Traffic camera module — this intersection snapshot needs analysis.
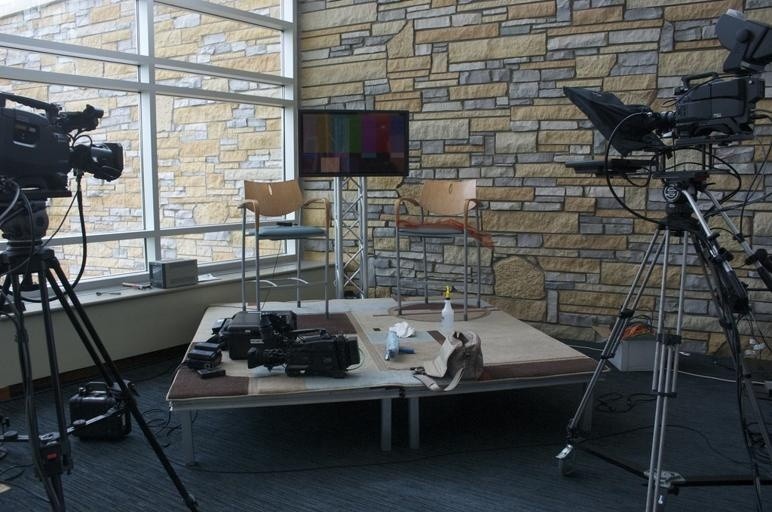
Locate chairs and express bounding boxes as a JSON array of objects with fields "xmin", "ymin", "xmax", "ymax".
[
  {"xmin": 238, "ymin": 179, "xmax": 331, "ymax": 319},
  {"xmin": 394, "ymin": 179, "xmax": 484, "ymax": 321}
]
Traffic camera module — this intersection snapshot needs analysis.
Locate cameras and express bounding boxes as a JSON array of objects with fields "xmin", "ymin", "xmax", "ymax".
[{"xmin": 188, "ymin": 342, "xmax": 223, "ymax": 370}]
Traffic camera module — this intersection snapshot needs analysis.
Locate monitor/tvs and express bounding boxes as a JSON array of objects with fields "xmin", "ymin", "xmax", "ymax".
[{"xmin": 298, "ymin": 109, "xmax": 410, "ymax": 178}]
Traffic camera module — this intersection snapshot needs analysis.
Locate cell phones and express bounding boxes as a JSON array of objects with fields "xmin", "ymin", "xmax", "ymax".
[{"xmin": 197, "ymin": 366, "xmax": 225, "ymax": 377}]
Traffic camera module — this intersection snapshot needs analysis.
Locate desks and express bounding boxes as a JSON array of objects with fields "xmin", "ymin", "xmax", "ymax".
[{"xmin": 165, "ymin": 293, "xmax": 610, "ymax": 451}]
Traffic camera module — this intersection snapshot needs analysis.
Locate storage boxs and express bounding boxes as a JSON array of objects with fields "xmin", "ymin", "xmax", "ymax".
[{"xmin": 591, "ymin": 324, "xmax": 665, "ymax": 372}]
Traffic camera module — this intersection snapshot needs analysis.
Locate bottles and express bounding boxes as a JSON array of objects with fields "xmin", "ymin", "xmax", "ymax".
[{"xmin": 442, "ymin": 298, "xmax": 455, "ymax": 334}]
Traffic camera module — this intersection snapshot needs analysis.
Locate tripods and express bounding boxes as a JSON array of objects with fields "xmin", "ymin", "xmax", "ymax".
[
  {"xmin": 0, "ymin": 246, "xmax": 199, "ymax": 512},
  {"xmin": 556, "ymin": 185, "xmax": 772, "ymax": 511}
]
